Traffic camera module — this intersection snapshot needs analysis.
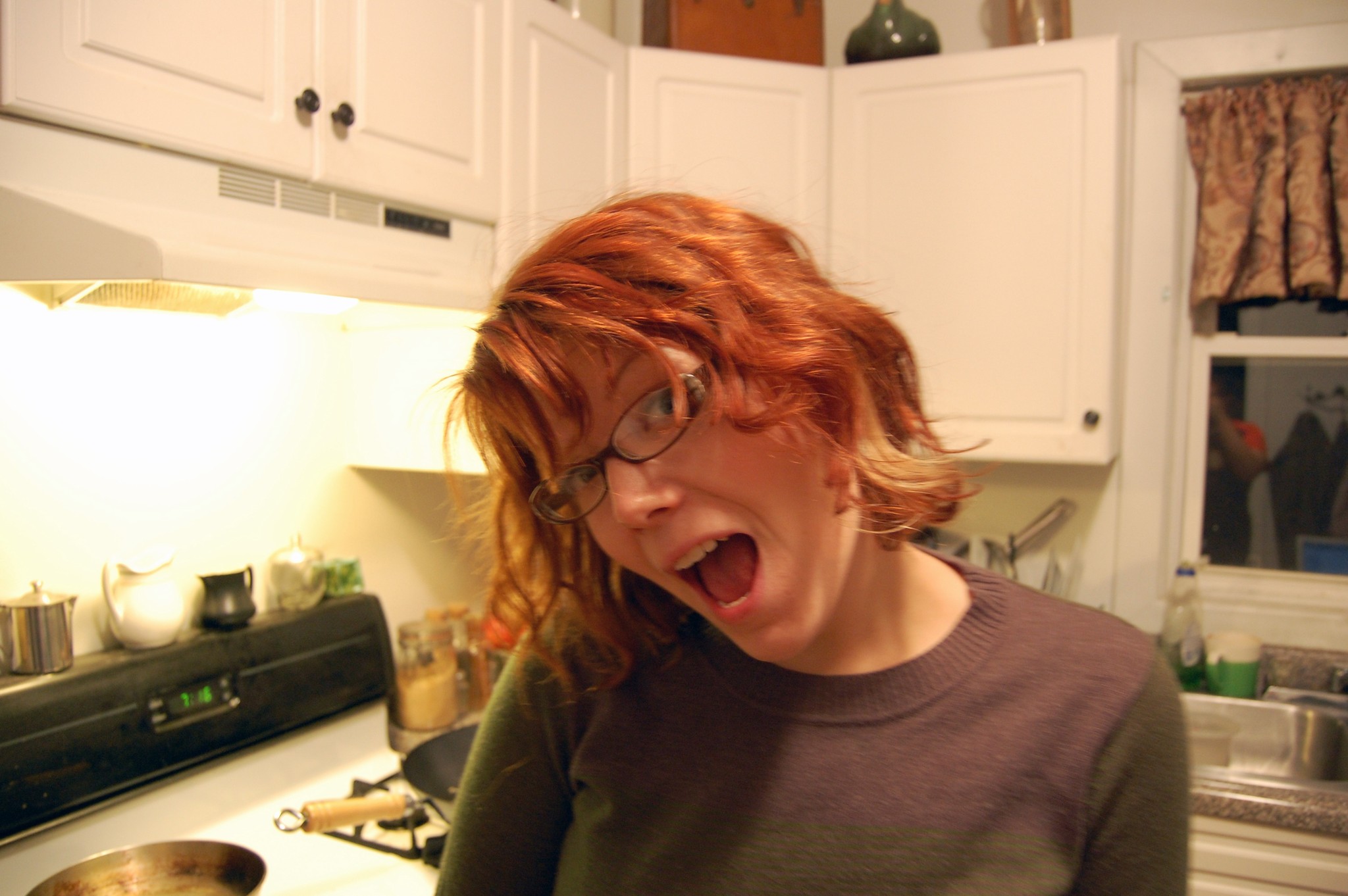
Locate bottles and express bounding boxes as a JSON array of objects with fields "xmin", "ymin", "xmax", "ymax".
[
  {"xmin": 846, "ymin": 0, "xmax": 941, "ymax": 65},
  {"xmin": 1159, "ymin": 564, "xmax": 1207, "ymax": 694}
]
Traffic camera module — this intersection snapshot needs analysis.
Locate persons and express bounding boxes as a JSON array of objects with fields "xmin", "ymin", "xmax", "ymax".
[{"xmin": 432, "ymin": 191, "xmax": 1193, "ymax": 896}]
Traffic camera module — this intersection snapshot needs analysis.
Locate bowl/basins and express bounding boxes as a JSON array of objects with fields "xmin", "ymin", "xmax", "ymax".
[{"xmin": 24, "ymin": 840, "xmax": 267, "ymax": 896}]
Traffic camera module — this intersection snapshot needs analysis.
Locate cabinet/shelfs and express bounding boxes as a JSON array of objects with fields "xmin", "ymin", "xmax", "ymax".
[
  {"xmin": 628, "ymin": 43, "xmax": 829, "ymax": 279},
  {"xmin": 1, "ymin": 1, "xmax": 500, "ymax": 225},
  {"xmin": 826, "ymin": 34, "xmax": 1109, "ymax": 469},
  {"xmin": 495, "ymin": 0, "xmax": 629, "ymax": 301}
]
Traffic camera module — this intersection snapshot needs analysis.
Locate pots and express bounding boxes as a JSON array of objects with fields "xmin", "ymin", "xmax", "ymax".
[{"xmin": 274, "ymin": 723, "xmax": 479, "ymax": 833}]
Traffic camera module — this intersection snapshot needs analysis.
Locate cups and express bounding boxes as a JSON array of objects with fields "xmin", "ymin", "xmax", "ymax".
[
  {"xmin": 1204, "ymin": 657, "xmax": 1263, "ymax": 701},
  {"xmin": 101, "ymin": 552, "xmax": 186, "ymax": 651},
  {"xmin": 1005, "ymin": 0, "xmax": 1072, "ymax": 45},
  {"xmin": 310, "ymin": 559, "xmax": 360, "ymax": 596},
  {"xmin": 199, "ymin": 564, "xmax": 257, "ymax": 629},
  {"xmin": 0, "ymin": 581, "xmax": 79, "ymax": 676}
]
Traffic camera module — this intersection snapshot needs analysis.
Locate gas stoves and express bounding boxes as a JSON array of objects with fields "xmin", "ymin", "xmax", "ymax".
[{"xmin": 0, "ymin": 591, "xmax": 451, "ymax": 896}]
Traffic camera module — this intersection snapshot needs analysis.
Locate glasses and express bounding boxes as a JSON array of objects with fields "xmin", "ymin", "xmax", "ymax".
[{"xmin": 529, "ymin": 356, "xmax": 725, "ymax": 527}]
